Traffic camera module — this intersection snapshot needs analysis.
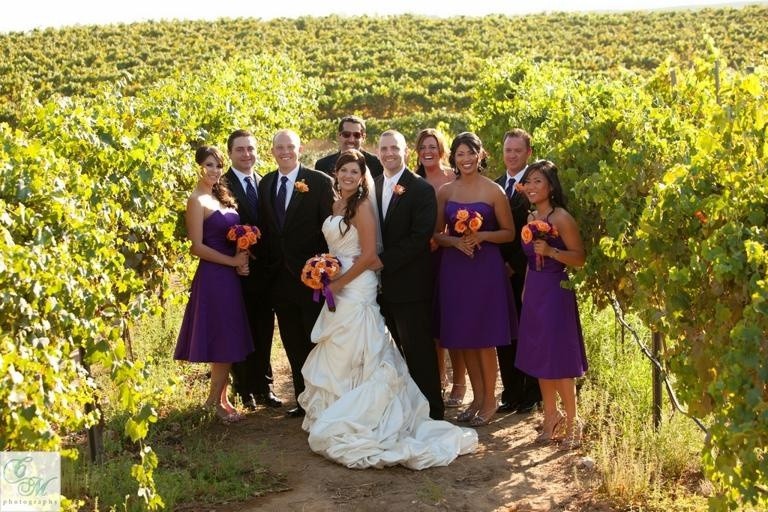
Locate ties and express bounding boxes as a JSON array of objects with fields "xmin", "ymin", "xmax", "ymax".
[
  {"xmin": 244, "ymin": 177, "xmax": 258, "ymax": 221},
  {"xmin": 276, "ymin": 176, "xmax": 288, "ymax": 230},
  {"xmin": 505, "ymin": 178, "xmax": 516, "ymax": 198}
]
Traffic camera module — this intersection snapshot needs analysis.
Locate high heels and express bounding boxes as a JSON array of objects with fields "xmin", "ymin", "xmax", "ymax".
[
  {"xmin": 470, "ymin": 400, "xmax": 499, "ymax": 427},
  {"xmin": 447, "ymin": 383, "xmax": 466, "ymax": 408},
  {"xmin": 558, "ymin": 419, "xmax": 582, "ymax": 450},
  {"xmin": 457, "ymin": 408, "xmax": 476, "ymax": 422},
  {"xmin": 440, "ymin": 375, "xmax": 448, "ymax": 397},
  {"xmin": 536, "ymin": 411, "xmax": 566, "ymax": 445}
]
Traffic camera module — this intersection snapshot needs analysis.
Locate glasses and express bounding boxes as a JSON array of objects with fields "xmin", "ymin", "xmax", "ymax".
[{"xmin": 339, "ymin": 130, "xmax": 363, "ymax": 139}]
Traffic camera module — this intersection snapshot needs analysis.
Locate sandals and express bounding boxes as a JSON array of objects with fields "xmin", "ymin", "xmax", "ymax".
[
  {"xmin": 204, "ymin": 402, "xmax": 238, "ymax": 423},
  {"xmin": 224, "ymin": 401, "xmax": 246, "ymax": 420}
]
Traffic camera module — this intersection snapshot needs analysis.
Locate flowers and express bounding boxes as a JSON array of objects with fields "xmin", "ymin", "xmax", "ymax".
[
  {"xmin": 453, "ymin": 208, "xmax": 486, "ymax": 236},
  {"xmin": 226, "ymin": 223, "xmax": 263, "ymax": 251},
  {"xmin": 300, "ymin": 252, "xmax": 342, "ymax": 292},
  {"xmin": 517, "ymin": 219, "xmax": 557, "ymax": 247}
]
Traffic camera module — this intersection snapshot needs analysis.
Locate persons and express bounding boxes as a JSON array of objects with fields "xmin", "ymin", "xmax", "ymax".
[{"xmin": 175, "ymin": 116, "xmax": 587, "ymax": 470}]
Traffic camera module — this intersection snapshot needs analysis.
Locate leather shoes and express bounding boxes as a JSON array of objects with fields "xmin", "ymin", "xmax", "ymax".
[
  {"xmin": 254, "ymin": 391, "xmax": 282, "ymax": 408},
  {"xmin": 285, "ymin": 406, "xmax": 305, "ymax": 418},
  {"xmin": 498, "ymin": 400, "xmax": 519, "ymax": 413},
  {"xmin": 239, "ymin": 392, "xmax": 257, "ymax": 410},
  {"xmin": 517, "ymin": 400, "xmax": 539, "ymax": 413}
]
